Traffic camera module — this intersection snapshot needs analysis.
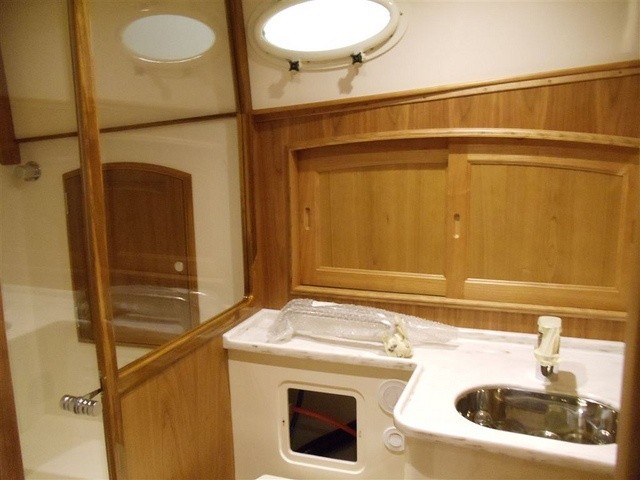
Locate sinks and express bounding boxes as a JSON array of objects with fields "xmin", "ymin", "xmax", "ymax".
[{"xmin": 453, "ymin": 382, "xmax": 619, "ymax": 447}]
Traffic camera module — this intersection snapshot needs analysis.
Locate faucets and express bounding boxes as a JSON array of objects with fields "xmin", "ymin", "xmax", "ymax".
[{"xmin": 533, "ymin": 316, "xmax": 562, "ymax": 386}]
